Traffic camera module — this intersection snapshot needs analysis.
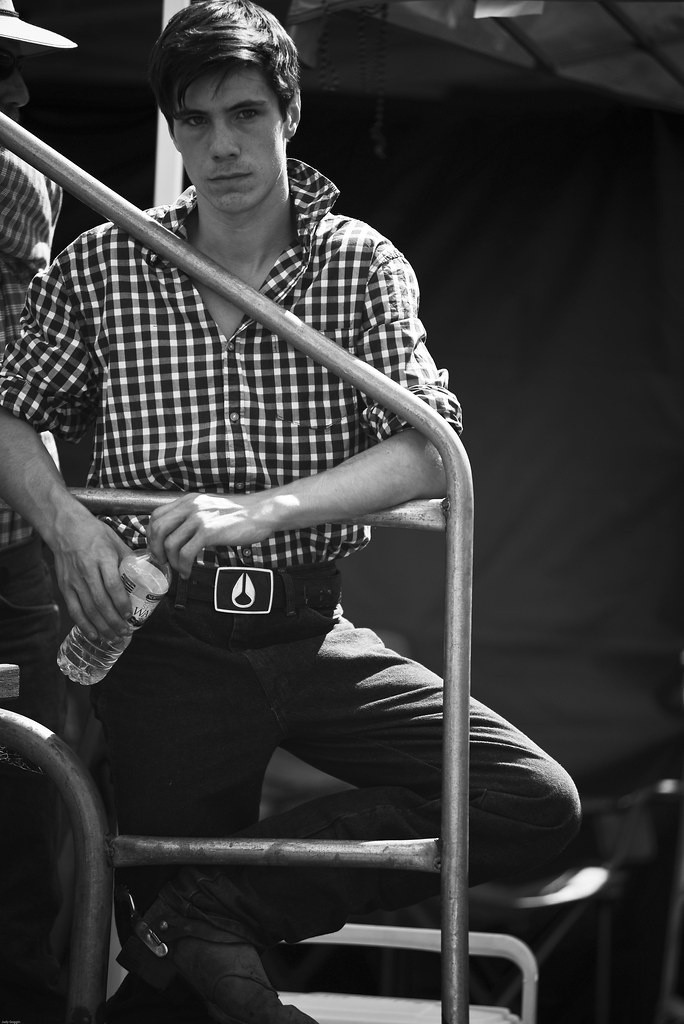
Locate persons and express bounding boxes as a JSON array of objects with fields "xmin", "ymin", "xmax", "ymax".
[
  {"xmin": 0, "ymin": 1, "xmax": 78, "ymax": 1024},
  {"xmin": 0, "ymin": 0, "xmax": 581, "ymax": 1024}
]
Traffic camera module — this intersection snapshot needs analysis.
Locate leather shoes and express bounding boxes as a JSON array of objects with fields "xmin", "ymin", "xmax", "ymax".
[{"xmin": 116, "ymin": 896, "xmax": 318, "ymax": 1024}]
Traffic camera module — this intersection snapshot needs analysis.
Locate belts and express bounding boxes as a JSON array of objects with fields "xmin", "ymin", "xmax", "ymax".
[{"xmin": 168, "ymin": 565, "xmax": 344, "ymax": 610}]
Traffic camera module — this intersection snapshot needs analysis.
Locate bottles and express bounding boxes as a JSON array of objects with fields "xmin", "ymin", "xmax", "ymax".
[{"xmin": 55, "ymin": 547, "xmax": 176, "ymax": 686}]
